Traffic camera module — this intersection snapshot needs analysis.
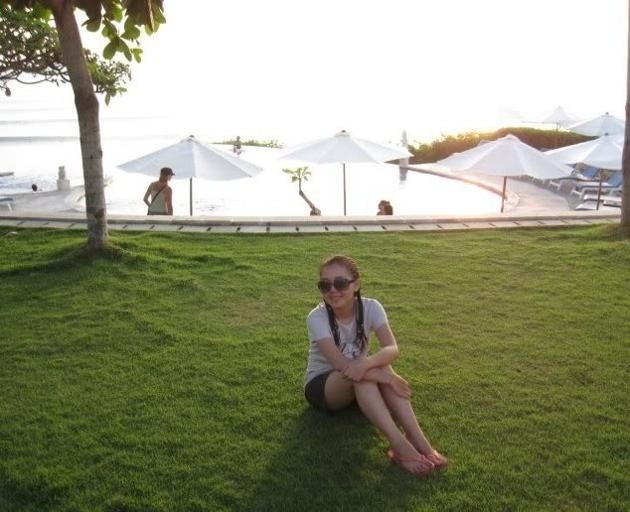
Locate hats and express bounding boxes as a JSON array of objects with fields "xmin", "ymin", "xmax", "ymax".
[{"xmin": 161, "ymin": 167, "xmax": 176, "ymax": 175}]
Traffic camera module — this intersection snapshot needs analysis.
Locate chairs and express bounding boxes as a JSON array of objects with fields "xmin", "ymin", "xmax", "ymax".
[{"xmin": 545, "ymin": 160, "xmax": 622, "ymax": 212}]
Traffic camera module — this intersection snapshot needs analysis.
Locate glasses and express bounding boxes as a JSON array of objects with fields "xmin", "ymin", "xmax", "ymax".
[{"xmin": 318, "ymin": 277, "xmax": 357, "ymax": 293}]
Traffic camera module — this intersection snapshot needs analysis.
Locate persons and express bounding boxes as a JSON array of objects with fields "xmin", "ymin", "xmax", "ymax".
[
  {"xmin": 377, "ymin": 200, "xmax": 390, "ymax": 215},
  {"xmin": 303, "ymin": 254, "xmax": 450, "ymax": 475},
  {"xmin": 144, "ymin": 166, "xmax": 174, "ymax": 216},
  {"xmin": 30, "ymin": 183, "xmax": 44, "ymax": 192},
  {"xmin": 382, "ymin": 205, "xmax": 393, "ymax": 215}
]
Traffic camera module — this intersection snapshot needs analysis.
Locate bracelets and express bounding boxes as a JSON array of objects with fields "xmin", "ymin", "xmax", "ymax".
[{"xmin": 387, "ymin": 372, "xmax": 396, "ymax": 384}]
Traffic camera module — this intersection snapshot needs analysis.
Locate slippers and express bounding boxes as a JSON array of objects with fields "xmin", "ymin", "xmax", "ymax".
[{"xmin": 387, "ymin": 439, "xmax": 446, "ymax": 476}]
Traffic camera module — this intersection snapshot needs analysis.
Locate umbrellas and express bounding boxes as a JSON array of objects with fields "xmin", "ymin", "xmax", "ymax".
[
  {"xmin": 116, "ymin": 134, "xmax": 264, "ymax": 216},
  {"xmin": 437, "ymin": 132, "xmax": 573, "ymax": 216},
  {"xmin": 277, "ymin": 129, "xmax": 415, "ymax": 215},
  {"xmin": 568, "ymin": 112, "xmax": 626, "ymax": 137},
  {"xmin": 543, "ymin": 131, "xmax": 623, "ymax": 209}
]
